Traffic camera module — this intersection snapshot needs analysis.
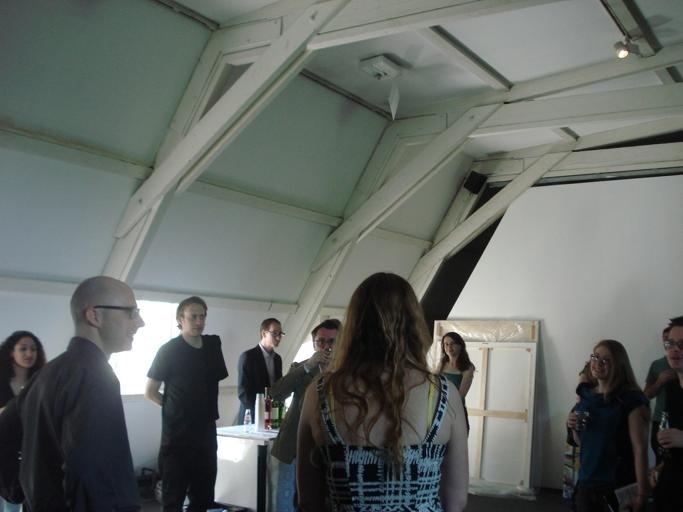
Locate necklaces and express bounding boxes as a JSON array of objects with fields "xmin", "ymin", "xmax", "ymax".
[{"xmin": 15, "ymin": 382, "xmax": 25, "ymax": 390}]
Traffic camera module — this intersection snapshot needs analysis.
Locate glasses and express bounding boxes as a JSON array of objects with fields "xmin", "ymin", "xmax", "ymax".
[
  {"xmin": 314, "ymin": 340, "xmax": 335, "ymax": 346},
  {"xmin": 662, "ymin": 341, "xmax": 683, "ymax": 350},
  {"xmin": 267, "ymin": 331, "xmax": 286, "ymax": 336},
  {"xmin": 92, "ymin": 304, "xmax": 142, "ymax": 319},
  {"xmin": 590, "ymin": 354, "xmax": 612, "ymax": 364}
]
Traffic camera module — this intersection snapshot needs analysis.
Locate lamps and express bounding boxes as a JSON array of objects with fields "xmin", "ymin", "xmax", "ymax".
[{"xmin": 613, "ymin": 41, "xmax": 629, "ymax": 59}]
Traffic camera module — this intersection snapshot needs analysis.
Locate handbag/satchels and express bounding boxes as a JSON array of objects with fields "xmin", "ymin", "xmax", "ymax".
[{"xmin": 136, "ymin": 467, "xmax": 160, "ymax": 500}]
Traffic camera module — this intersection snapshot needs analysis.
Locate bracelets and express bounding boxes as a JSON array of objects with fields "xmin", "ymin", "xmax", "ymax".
[{"xmin": 635, "ymin": 494, "xmax": 648, "ymax": 497}]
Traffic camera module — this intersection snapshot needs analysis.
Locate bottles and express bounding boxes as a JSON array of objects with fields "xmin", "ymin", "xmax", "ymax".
[
  {"xmin": 657, "ymin": 411, "xmax": 670, "ymax": 456},
  {"xmin": 272, "ymin": 401, "xmax": 280, "ymax": 428},
  {"xmin": 318, "ymin": 348, "xmax": 332, "ymax": 372},
  {"xmin": 264, "ymin": 387, "xmax": 273, "ymax": 430},
  {"xmin": 255, "ymin": 394, "xmax": 265, "ymax": 432},
  {"xmin": 244, "ymin": 409, "xmax": 252, "ymax": 435},
  {"xmin": 281, "ymin": 403, "xmax": 287, "ymax": 428}
]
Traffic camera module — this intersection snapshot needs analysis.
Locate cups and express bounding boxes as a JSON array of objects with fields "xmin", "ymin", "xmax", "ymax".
[{"xmin": 575, "ymin": 411, "xmax": 589, "ymax": 431}]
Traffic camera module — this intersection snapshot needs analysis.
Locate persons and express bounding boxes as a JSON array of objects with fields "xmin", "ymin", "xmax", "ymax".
[
  {"xmin": 144, "ymin": 296, "xmax": 229, "ymax": 512},
  {"xmin": 567, "ymin": 340, "xmax": 651, "ymax": 512},
  {"xmin": 643, "ymin": 328, "xmax": 679, "ymax": 466},
  {"xmin": 297, "ymin": 272, "xmax": 469, "ymax": 512},
  {"xmin": 0, "ymin": 276, "xmax": 145, "ymax": 512},
  {"xmin": 232, "ymin": 316, "xmax": 286, "ymax": 424},
  {"xmin": 434, "ymin": 332, "xmax": 475, "ymax": 438},
  {"xmin": 271, "ymin": 319, "xmax": 343, "ymax": 512},
  {"xmin": 649, "ymin": 317, "xmax": 683, "ymax": 512},
  {"xmin": 0, "ymin": 330, "xmax": 48, "ymax": 512}
]
